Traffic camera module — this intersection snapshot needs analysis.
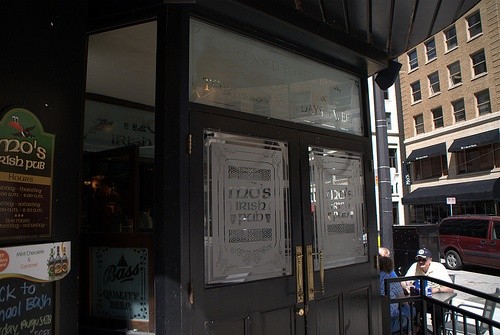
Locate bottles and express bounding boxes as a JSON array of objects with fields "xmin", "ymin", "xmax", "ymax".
[
  {"xmin": 62, "ymin": 246, "xmax": 68, "ymax": 273},
  {"xmin": 407, "ymin": 281, "xmax": 432, "ymax": 298},
  {"xmin": 54, "ymin": 246, "xmax": 63, "ymax": 276},
  {"xmin": 47, "ymin": 247, "xmax": 56, "ymax": 279}
]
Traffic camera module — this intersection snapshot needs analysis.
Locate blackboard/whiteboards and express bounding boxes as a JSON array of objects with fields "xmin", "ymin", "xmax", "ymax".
[{"xmin": 0, "ymin": 274, "xmax": 60, "ymax": 335}]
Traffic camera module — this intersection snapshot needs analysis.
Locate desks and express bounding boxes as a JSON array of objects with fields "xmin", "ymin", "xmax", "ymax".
[{"xmin": 431, "ymin": 293, "xmax": 457, "ymax": 335}]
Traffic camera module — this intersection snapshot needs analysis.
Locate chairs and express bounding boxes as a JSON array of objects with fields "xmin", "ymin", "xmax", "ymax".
[{"xmin": 441, "ymin": 288, "xmax": 500, "ymax": 335}]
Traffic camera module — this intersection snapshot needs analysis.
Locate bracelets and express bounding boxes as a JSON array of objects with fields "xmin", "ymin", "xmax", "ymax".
[{"xmin": 439, "ymin": 287, "xmax": 440, "ymax": 292}]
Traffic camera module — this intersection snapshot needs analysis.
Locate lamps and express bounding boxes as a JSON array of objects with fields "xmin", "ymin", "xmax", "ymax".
[{"xmin": 375, "ymin": 59, "xmax": 403, "ymax": 90}]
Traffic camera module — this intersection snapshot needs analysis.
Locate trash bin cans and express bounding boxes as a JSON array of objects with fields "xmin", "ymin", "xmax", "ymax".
[{"xmin": 392, "ymin": 224, "xmax": 440, "ymax": 277}]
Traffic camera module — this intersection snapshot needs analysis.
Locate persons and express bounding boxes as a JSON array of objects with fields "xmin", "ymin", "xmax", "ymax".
[
  {"xmin": 404, "ymin": 247, "xmax": 454, "ymax": 335},
  {"xmin": 379, "ymin": 248, "xmax": 416, "ymax": 319}
]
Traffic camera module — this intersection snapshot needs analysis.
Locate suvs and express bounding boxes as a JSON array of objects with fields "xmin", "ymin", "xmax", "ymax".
[{"xmin": 437, "ymin": 214, "xmax": 500, "ymax": 271}]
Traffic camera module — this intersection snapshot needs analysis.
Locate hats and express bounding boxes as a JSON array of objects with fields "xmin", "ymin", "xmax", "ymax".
[{"xmin": 415, "ymin": 248, "xmax": 430, "ymax": 258}]
